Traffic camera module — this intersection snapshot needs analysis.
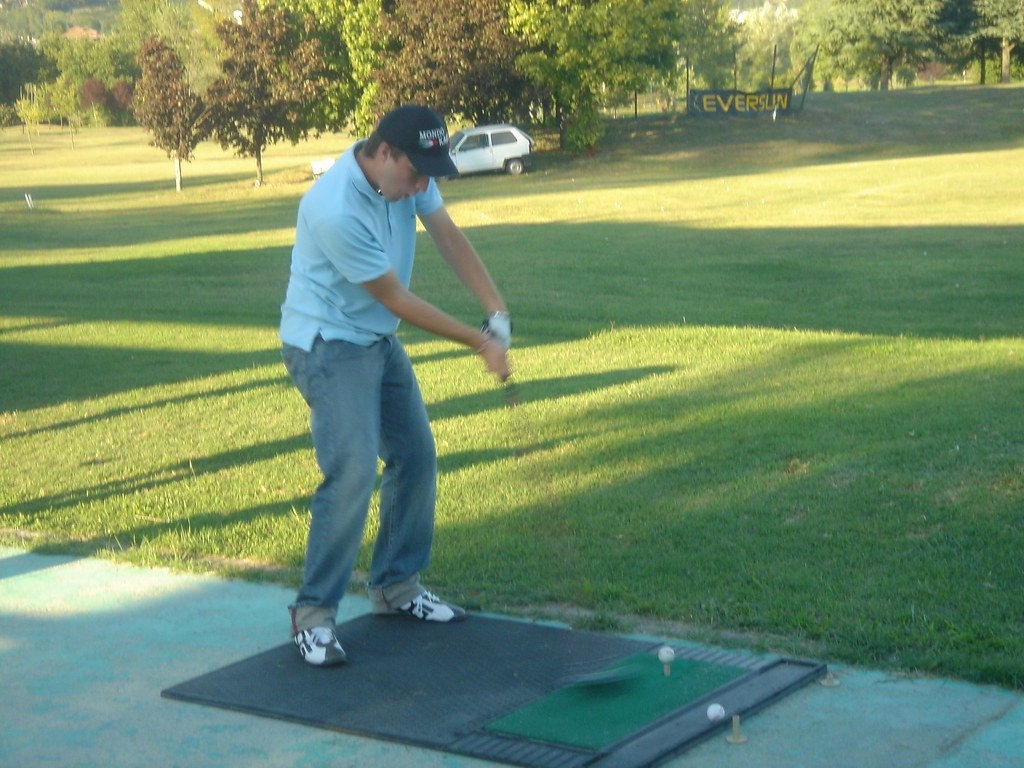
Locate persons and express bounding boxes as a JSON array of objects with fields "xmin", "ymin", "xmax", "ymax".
[{"xmin": 279, "ymin": 102, "xmax": 513, "ymax": 671}]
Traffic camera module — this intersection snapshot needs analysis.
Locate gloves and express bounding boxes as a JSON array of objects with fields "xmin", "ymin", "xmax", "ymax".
[{"xmin": 488, "ymin": 310, "xmax": 511, "ymax": 348}]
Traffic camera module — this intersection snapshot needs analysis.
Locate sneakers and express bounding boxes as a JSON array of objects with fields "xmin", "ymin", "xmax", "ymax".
[
  {"xmin": 293, "ymin": 626, "xmax": 346, "ymax": 665},
  {"xmin": 391, "ymin": 591, "xmax": 464, "ymax": 622}
]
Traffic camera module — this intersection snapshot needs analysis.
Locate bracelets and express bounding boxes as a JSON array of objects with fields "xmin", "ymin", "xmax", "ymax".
[
  {"xmin": 475, "ymin": 336, "xmax": 492, "ymax": 357},
  {"xmin": 487, "ymin": 310, "xmax": 511, "ymax": 318}
]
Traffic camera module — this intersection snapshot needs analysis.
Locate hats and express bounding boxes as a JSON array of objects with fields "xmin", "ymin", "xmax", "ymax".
[{"xmin": 378, "ymin": 105, "xmax": 458, "ymax": 176}]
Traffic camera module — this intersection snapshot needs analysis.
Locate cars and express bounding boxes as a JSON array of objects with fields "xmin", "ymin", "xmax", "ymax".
[{"xmin": 440, "ymin": 124, "xmax": 538, "ymax": 181}]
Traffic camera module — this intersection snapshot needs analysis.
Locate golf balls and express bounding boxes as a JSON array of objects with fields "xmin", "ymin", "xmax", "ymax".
[
  {"xmin": 657, "ymin": 646, "xmax": 675, "ymax": 663},
  {"xmin": 706, "ymin": 702, "xmax": 725, "ymax": 722}
]
